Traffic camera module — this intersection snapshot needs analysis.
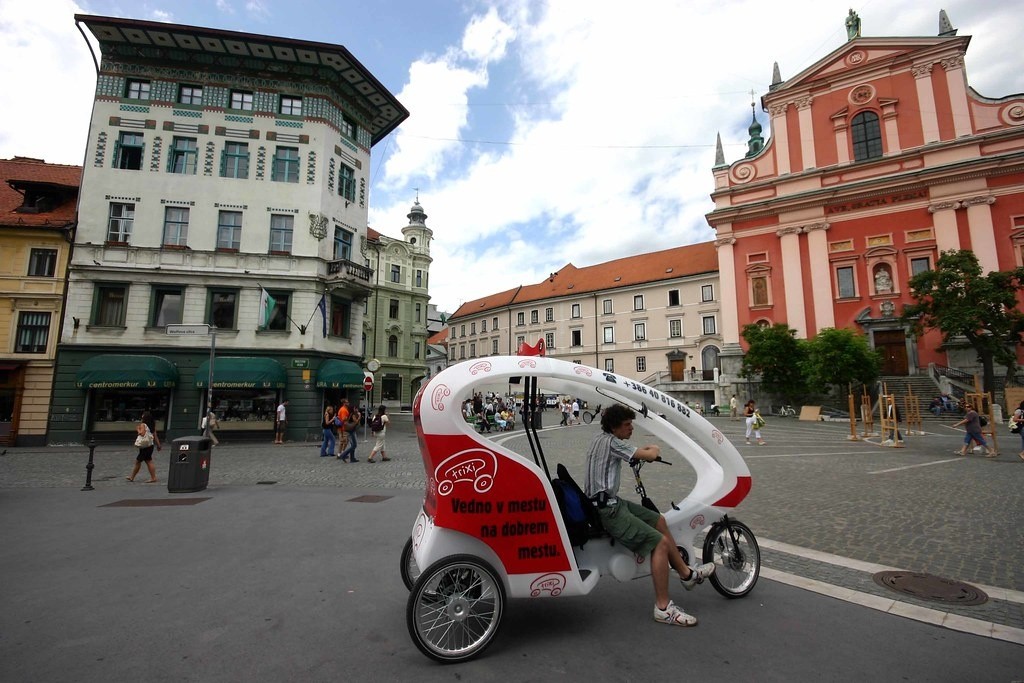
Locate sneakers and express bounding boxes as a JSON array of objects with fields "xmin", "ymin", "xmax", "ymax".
[
  {"xmin": 680, "ymin": 563, "xmax": 715, "ymax": 591},
  {"xmin": 654, "ymin": 600, "xmax": 697, "ymax": 626}
]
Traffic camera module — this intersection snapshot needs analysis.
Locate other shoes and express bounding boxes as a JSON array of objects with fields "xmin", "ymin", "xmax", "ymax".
[
  {"xmin": 746, "ymin": 442, "xmax": 751, "ymax": 444},
  {"xmin": 382, "ymin": 457, "xmax": 390, "ymax": 461},
  {"xmin": 328, "ymin": 453, "xmax": 336, "ymax": 456},
  {"xmin": 339, "ymin": 455, "xmax": 347, "ymax": 463},
  {"xmin": 985, "ymin": 452, "xmax": 997, "ymax": 457},
  {"xmin": 898, "ymin": 440, "xmax": 903, "ymax": 442},
  {"xmin": 885, "ymin": 439, "xmax": 893, "ymax": 443},
  {"xmin": 350, "ymin": 458, "xmax": 359, "ymax": 462},
  {"xmin": 145, "ymin": 478, "xmax": 155, "ymax": 482},
  {"xmin": 954, "ymin": 451, "xmax": 966, "ymax": 456},
  {"xmin": 337, "ymin": 453, "xmax": 349, "ymax": 459},
  {"xmin": 367, "ymin": 457, "xmax": 375, "ymax": 463},
  {"xmin": 1018, "ymin": 453, "xmax": 1024, "ymax": 460},
  {"xmin": 126, "ymin": 478, "xmax": 133, "ymax": 481},
  {"xmin": 759, "ymin": 442, "xmax": 766, "ymax": 445}
]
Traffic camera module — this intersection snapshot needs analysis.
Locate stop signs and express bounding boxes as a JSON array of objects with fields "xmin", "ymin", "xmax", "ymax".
[{"xmin": 364, "ymin": 376, "xmax": 373, "ymax": 391}]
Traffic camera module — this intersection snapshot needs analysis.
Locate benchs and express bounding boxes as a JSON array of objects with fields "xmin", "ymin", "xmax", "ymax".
[
  {"xmin": 464, "ymin": 415, "xmax": 501, "ymax": 432},
  {"xmin": 703, "ymin": 411, "xmax": 716, "ymax": 417}
]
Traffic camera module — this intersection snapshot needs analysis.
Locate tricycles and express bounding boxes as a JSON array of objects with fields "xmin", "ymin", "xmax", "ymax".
[{"xmin": 402, "ymin": 337, "xmax": 762, "ymax": 664}]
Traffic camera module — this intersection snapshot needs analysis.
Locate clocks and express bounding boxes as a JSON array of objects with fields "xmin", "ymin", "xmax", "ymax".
[
  {"xmin": 366, "ymin": 358, "xmax": 381, "ymax": 373},
  {"xmin": 848, "ymin": 83, "xmax": 876, "ymax": 106}
]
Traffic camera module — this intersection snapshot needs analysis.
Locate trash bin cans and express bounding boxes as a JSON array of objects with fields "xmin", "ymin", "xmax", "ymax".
[
  {"xmin": 532, "ymin": 412, "xmax": 542, "ymax": 429},
  {"xmin": 992, "ymin": 403, "xmax": 1003, "ymax": 423},
  {"xmin": 168, "ymin": 436, "xmax": 212, "ymax": 492}
]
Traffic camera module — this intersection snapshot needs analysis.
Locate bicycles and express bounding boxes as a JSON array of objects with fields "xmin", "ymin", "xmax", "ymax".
[{"xmin": 777, "ymin": 404, "xmax": 796, "ymax": 418}]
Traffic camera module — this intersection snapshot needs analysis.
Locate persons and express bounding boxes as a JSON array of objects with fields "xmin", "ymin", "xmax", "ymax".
[
  {"xmin": 463, "ymin": 391, "xmax": 585, "ymax": 433},
  {"xmin": 730, "ymin": 395, "xmax": 740, "ymax": 421},
  {"xmin": 274, "ymin": 399, "xmax": 290, "ymax": 445},
  {"xmin": 929, "ymin": 396, "xmax": 966, "ymax": 416},
  {"xmin": 584, "ymin": 404, "xmax": 716, "ymax": 627},
  {"xmin": 126, "ymin": 411, "xmax": 161, "ymax": 483},
  {"xmin": 953, "ymin": 402, "xmax": 997, "ymax": 458},
  {"xmin": 202, "ymin": 407, "xmax": 220, "ymax": 448},
  {"xmin": 883, "ymin": 398, "xmax": 904, "ymax": 442},
  {"xmin": 320, "ymin": 398, "xmax": 391, "ymax": 464},
  {"xmin": 875, "ymin": 267, "xmax": 893, "ymax": 291},
  {"xmin": 1013, "ymin": 400, "xmax": 1024, "ymax": 460},
  {"xmin": 743, "ymin": 400, "xmax": 767, "ymax": 445},
  {"xmin": 684, "ymin": 401, "xmax": 689, "ymax": 407}
]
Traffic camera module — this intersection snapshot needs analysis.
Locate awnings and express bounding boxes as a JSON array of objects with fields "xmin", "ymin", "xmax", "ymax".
[
  {"xmin": 194, "ymin": 355, "xmax": 288, "ymax": 390},
  {"xmin": 74, "ymin": 355, "xmax": 180, "ymax": 390},
  {"xmin": 316, "ymin": 358, "xmax": 366, "ymax": 390}
]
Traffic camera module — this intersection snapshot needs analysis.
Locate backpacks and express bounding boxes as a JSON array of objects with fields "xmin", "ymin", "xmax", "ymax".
[
  {"xmin": 371, "ymin": 413, "xmax": 385, "ymax": 434},
  {"xmin": 551, "ymin": 462, "xmax": 604, "ymax": 546}
]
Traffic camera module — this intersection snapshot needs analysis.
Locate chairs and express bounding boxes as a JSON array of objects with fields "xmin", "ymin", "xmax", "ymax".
[{"xmin": 556, "ymin": 461, "xmax": 605, "ymax": 540}]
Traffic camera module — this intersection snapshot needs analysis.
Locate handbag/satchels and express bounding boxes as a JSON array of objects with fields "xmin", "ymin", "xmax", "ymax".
[
  {"xmin": 202, "ymin": 417, "xmax": 216, "ymax": 429},
  {"xmin": 569, "ymin": 412, "xmax": 576, "ymax": 421},
  {"xmin": 1008, "ymin": 408, "xmax": 1023, "ymax": 434},
  {"xmin": 751, "ymin": 412, "xmax": 766, "ymax": 429},
  {"xmin": 134, "ymin": 435, "xmax": 150, "ymax": 449},
  {"xmin": 333, "ymin": 417, "xmax": 342, "ymax": 428},
  {"xmin": 979, "ymin": 416, "xmax": 987, "ymax": 427},
  {"xmin": 344, "ymin": 413, "xmax": 359, "ymax": 432},
  {"xmin": 322, "ymin": 422, "xmax": 330, "ymax": 429}
]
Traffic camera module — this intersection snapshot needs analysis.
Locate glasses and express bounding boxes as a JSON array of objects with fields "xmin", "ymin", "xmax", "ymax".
[{"xmin": 964, "ymin": 406, "xmax": 971, "ymax": 408}]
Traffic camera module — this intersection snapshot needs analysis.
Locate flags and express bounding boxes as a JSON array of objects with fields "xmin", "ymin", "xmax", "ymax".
[
  {"xmin": 318, "ymin": 292, "xmax": 328, "ymax": 338},
  {"xmin": 440, "ymin": 312, "xmax": 446, "ymax": 326},
  {"xmin": 258, "ymin": 289, "xmax": 276, "ymax": 329}
]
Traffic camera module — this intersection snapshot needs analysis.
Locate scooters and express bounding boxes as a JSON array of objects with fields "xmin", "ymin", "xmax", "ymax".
[{"xmin": 361, "ymin": 404, "xmax": 375, "ymax": 426}]
